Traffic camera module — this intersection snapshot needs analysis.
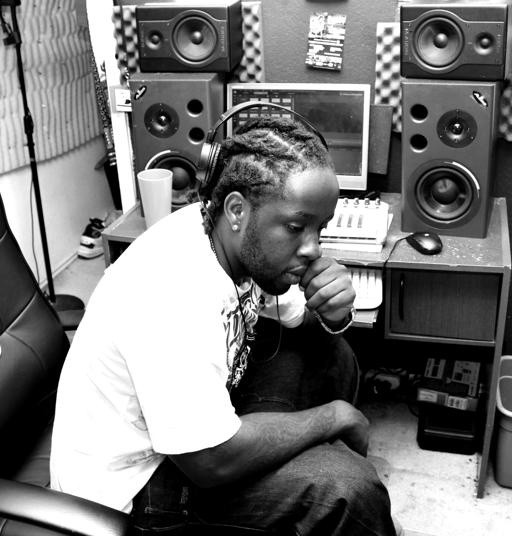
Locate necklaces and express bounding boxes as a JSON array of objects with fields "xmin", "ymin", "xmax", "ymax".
[{"xmin": 208, "ymin": 233, "xmax": 221, "ymax": 262}]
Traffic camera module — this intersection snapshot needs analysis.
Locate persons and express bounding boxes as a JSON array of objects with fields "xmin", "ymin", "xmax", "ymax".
[{"xmin": 48, "ymin": 110, "xmax": 399, "ymax": 536}]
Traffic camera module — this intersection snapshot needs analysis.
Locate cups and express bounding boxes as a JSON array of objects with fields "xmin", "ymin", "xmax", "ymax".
[{"xmin": 137, "ymin": 168, "xmax": 173, "ymax": 230}]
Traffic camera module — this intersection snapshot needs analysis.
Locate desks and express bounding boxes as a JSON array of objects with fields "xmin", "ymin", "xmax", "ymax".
[{"xmin": 100, "ymin": 194, "xmax": 511, "ymax": 498}]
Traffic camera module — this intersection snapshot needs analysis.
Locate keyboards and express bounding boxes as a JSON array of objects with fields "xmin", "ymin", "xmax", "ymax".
[{"xmin": 348, "ymin": 266, "xmax": 383, "ymax": 311}]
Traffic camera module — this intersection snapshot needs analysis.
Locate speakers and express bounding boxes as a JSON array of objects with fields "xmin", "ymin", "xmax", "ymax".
[
  {"xmin": 130, "ymin": 72, "xmax": 223, "ymax": 217},
  {"xmin": 402, "ymin": 82, "xmax": 501, "ymax": 239},
  {"xmin": 401, "ymin": 5, "xmax": 507, "ymax": 82},
  {"xmin": 136, "ymin": 0, "xmax": 243, "ymax": 73}
]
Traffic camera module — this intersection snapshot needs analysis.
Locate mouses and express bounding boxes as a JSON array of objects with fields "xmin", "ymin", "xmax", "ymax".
[{"xmin": 408, "ymin": 231, "xmax": 443, "ymax": 254}]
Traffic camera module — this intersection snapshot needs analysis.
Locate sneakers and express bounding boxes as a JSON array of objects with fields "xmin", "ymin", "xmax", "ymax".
[{"xmin": 77, "ymin": 218, "xmax": 107, "ymax": 259}]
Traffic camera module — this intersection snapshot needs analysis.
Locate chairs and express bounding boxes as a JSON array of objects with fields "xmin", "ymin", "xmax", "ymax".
[{"xmin": 0, "ymin": 193, "xmax": 134, "ymax": 535}]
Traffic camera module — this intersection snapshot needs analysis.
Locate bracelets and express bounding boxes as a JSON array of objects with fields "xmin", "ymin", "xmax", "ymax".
[{"xmin": 311, "ymin": 307, "xmax": 358, "ymax": 337}]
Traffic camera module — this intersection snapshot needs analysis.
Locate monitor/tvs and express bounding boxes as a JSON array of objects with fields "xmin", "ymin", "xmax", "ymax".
[{"xmin": 227, "ymin": 83, "xmax": 371, "ymax": 192}]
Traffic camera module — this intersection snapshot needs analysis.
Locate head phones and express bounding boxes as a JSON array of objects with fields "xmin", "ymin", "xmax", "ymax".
[{"xmin": 187, "ymin": 100, "xmax": 328, "ymax": 194}]
